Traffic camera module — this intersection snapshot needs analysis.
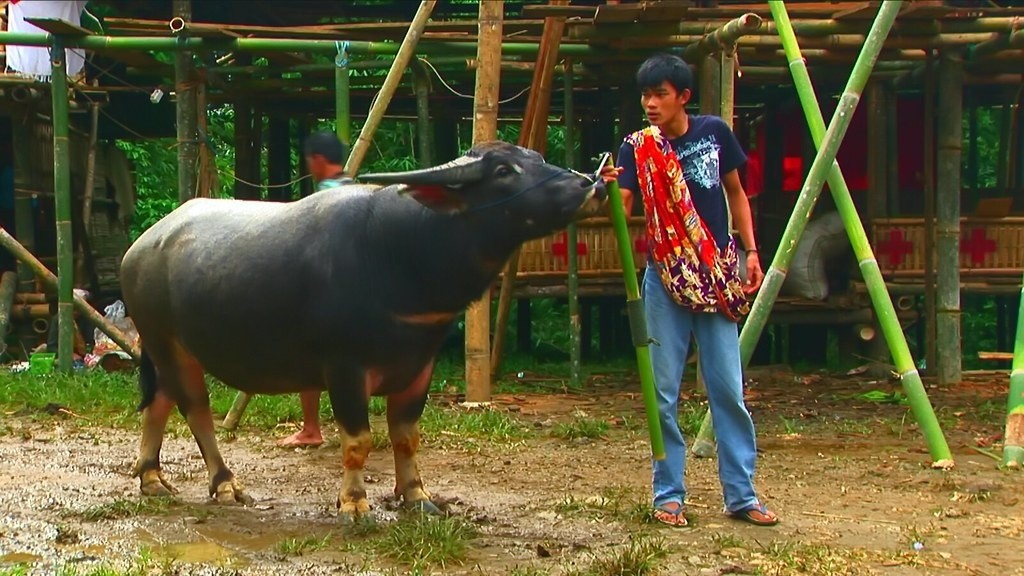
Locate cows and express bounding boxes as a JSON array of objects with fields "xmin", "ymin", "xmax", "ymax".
[{"xmin": 120, "ymin": 141, "xmax": 608, "ymax": 538}]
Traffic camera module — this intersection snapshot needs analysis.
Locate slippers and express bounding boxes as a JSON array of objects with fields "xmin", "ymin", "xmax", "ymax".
[
  {"xmin": 652, "ymin": 502, "xmax": 689, "ymax": 526},
  {"xmin": 732, "ymin": 504, "xmax": 779, "ymax": 525}
]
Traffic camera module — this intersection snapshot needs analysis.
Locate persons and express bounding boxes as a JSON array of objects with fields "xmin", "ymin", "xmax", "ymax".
[
  {"xmin": 276, "ymin": 130, "xmax": 358, "ymax": 447},
  {"xmin": 595, "ymin": 55, "xmax": 778, "ymax": 527}
]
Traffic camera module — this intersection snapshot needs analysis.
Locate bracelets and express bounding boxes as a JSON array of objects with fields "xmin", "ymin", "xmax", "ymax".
[{"xmin": 746, "ymin": 250, "xmax": 758, "ymax": 254}]
